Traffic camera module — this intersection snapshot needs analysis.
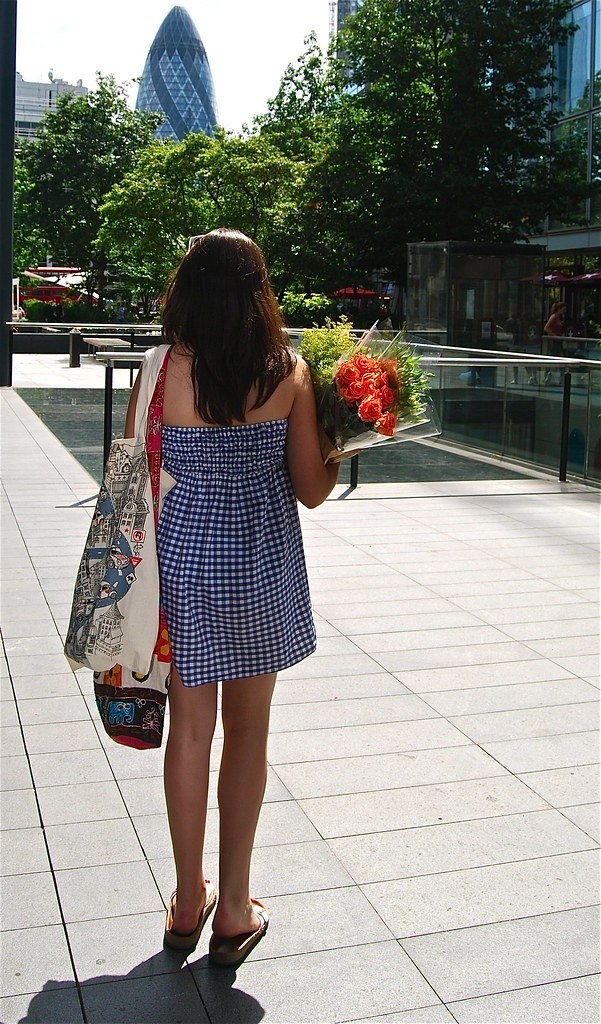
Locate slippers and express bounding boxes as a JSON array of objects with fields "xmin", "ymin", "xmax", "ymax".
[
  {"xmin": 209, "ymin": 898, "xmax": 269, "ymax": 967},
  {"xmin": 165, "ymin": 880, "xmax": 216, "ymax": 949}
]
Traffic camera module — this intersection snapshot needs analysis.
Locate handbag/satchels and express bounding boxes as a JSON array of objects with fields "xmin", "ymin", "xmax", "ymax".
[
  {"xmin": 93, "ymin": 608, "xmax": 172, "ymax": 750},
  {"xmin": 64, "ymin": 344, "xmax": 171, "ymax": 675}
]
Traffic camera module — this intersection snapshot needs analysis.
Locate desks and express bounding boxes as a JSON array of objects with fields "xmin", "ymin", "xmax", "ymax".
[{"xmin": 83, "ymin": 338, "xmax": 136, "ymax": 362}]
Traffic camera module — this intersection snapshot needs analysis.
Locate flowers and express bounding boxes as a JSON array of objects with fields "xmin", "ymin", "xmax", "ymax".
[{"xmin": 298, "ymin": 316, "xmax": 437, "ymax": 451}]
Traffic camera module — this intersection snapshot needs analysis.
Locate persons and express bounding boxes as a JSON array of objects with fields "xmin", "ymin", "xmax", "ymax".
[
  {"xmin": 126, "ymin": 227, "xmax": 338, "ymax": 968},
  {"xmin": 115, "ymin": 302, "xmax": 124, "ymax": 322},
  {"xmin": 544, "ymin": 301, "xmax": 568, "ymax": 356}
]
